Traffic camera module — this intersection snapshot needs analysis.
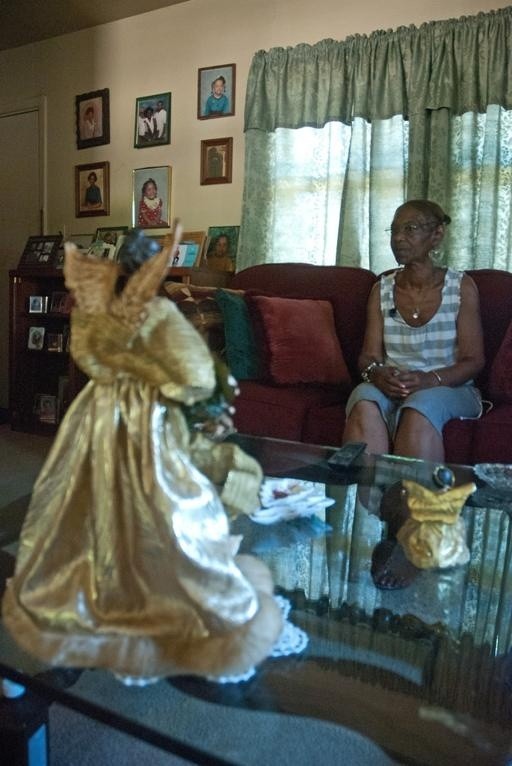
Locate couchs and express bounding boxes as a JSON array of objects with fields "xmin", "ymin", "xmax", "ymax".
[{"xmin": 209, "ymin": 264, "xmax": 511, "ymax": 465}]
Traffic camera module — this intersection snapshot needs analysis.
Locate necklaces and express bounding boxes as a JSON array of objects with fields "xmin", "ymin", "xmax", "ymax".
[{"xmin": 401, "ymin": 266, "xmax": 435, "ymax": 319}]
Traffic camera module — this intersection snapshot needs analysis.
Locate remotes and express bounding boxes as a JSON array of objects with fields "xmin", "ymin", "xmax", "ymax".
[{"xmin": 327, "ymin": 441, "xmax": 367, "ymax": 471}]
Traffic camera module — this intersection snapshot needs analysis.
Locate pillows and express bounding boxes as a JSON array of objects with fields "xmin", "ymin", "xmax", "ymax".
[
  {"xmin": 242, "ymin": 289, "xmax": 361, "ymax": 388},
  {"xmin": 212, "ymin": 288, "xmax": 259, "ymax": 381}
]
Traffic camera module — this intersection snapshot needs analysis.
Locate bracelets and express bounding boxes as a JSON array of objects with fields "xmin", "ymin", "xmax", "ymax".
[
  {"xmin": 359, "ymin": 360, "xmax": 385, "ymax": 385},
  {"xmin": 428, "ymin": 368, "xmax": 443, "ymax": 387}
]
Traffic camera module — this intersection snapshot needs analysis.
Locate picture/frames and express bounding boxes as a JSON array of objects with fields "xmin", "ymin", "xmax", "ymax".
[
  {"xmin": 134, "ymin": 92, "xmax": 171, "ymax": 148},
  {"xmin": 74, "ymin": 161, "xmax": 110, "ymax": 218},
  {"xmin": 75, "ymin": 88, "xmax": 110, "ymax": 150},
  {"xmin": 200, "ymin": 137, "xmax": 233, "ymax": 185},
  {"xmin": 197, "ymin": 63, "xmax": 235, "ymax": 120},
  {"xmin": 131, "ymin": 166, "xmax": 172, "ymax": 229}
]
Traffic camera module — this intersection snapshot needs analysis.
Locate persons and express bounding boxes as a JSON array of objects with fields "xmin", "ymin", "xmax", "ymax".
[
  {"xmin": 82, "ymin": 170, "xmax": 104, "ymax": 209},
  {"xmin": 172, "ymin": 244, "xmax": 181, "ymax": 265},
  {"xmin": 139, "ymin": 107, "xmax": 145, "ymax": 143},
  {"xmin": 138, "ymin": 176, "xmax": 168, "ymax": 228},
  {"xmin": 340, "ymin": 198, "xmax": 486, "ymax": 537},
  {"xmin": 204, "ymin": 75, "xmax": 231, "ymax": 118},
  {"xmin": 153, "ymin": 100, "xmax": 167, "ymax": 138},
  {"xmin": 144, "ymin": 106, "xmax": 158, "ymax": 141},
  {"xmin": 82, "ymin": 106, "xmax": 101, "ymax": 140},
  {"xmin": 205, "ymin": 232, "xmax": 236, "ymax": 272},
  {"xmin": 356, "ymin": 458, "xmax": 448, "ymax": 592},
  {"xmin": 41, "ymin": 398, "xmax": 54, "ymax": 415}
]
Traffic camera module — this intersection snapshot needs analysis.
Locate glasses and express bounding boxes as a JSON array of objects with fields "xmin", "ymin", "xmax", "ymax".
[{"xmin": 384, "ymin": 223, "xmax": 436, "ymax": 236}]
